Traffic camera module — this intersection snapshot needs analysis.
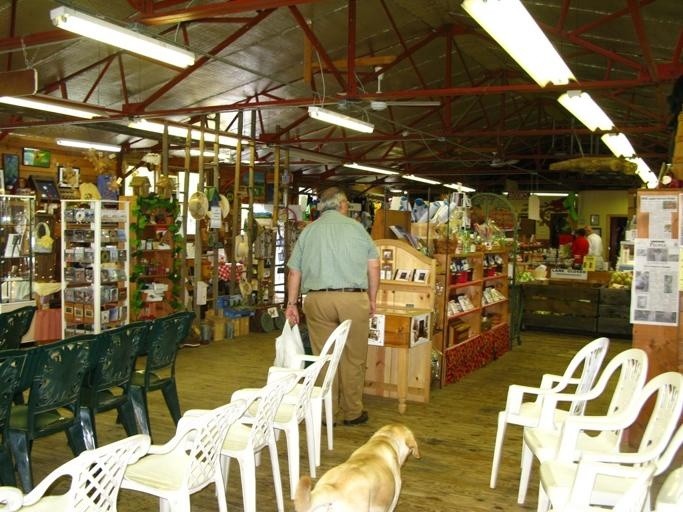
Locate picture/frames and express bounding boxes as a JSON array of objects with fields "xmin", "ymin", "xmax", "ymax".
[
  {"xmin": 590, "ymin": 214, "xmax": 600, "ymax": 225},
  {"xmin": 1, "ymin": 145, "xmax": 81, "ymax": 203},
  {"xmin": 590, "ymin": 227, "xmax": 602, "ymax": 237}
]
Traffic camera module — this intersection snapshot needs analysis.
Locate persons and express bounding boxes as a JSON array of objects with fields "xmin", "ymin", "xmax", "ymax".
[
  {"xmin": 570, "ymin": 229, "xmax": 588, "ymax": 266},
  {"xmin": 583, "ymin": 225, "xmax": 603, "ymax": 257},
  {"xmin": 284, "ymin": 186, "xmax": 380, "ymax": 424}
]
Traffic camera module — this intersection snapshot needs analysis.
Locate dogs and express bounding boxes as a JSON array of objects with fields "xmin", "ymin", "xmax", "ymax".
[{"xmin": 294, "ymin": 422, "xmax": 421, "ymax": 512}]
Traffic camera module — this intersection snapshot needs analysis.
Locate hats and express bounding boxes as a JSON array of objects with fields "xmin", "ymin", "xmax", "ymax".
[
  {"xmin": 189, "ymin": 192, "xmax": 208, "ymax": 220},
  {"xmin": 207, "ymin": 194, "xmax": 230, "ymax": 219},
  {"xmin": 235, "ymin": 235, "xmax": 249, "ymax": 263},
  {"xmin": 239, "ymin": 280, "xmax": 252, "ymax": 297}
]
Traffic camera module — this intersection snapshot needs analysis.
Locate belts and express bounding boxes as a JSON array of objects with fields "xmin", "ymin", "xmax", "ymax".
[{"xmin": 309, "ymin": 289, "xmax": 366, "ymax": 292}]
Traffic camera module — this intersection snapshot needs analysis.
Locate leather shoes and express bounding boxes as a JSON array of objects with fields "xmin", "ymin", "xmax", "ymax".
[
  {"xmin": 321, "ymin": 420, "xmax": 337, "ymax": 426},
  {"xmin": 345, "ymin": 411, "xmax": 368, "ymax": 425}
]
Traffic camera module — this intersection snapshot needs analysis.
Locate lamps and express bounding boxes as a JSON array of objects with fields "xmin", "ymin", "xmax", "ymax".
[
  {"xmin": 48, "ymin": 3, "xmax": 198, "ymax": 71},
  {"xmin": 600, "ymin": 133, "xmax": 662, "ymax": 191},
  {"xmin": 307, "ymin": 103, "xmax": 376, "ymax": 136},
  {"xmin": 459, "ymin": 0, "xmax": 577, "ymax": 88},
  {"xmin": 557, "ymin": 92, "xmax": 620, "ymax": 134},
  {"xmin": 341, "ymin": 160, "xmax": 475, "ymax": 196}
]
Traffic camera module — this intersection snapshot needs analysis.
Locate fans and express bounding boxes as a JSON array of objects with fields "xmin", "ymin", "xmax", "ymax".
[
  {"xmin": 337, "ymin": 65, "xmax": 442, "ymax": 112},
  {"xmin": 475, "ymin": 148, "xmax": 520, "ymax": 169}
]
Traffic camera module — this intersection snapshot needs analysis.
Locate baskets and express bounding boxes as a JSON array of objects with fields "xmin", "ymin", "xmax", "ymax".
[
  {"xmin": 433, "ymin": 238, "xmax": 457, "ymax": 254},
  {"xmin": 34, "ymin": 222, "xmax": 53, "ymax": 253},
  {"xmin": 56, "ymin": 180, "xmax": 80, "ymax": 199}
]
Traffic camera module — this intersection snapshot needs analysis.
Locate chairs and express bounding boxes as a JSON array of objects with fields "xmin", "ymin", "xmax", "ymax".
[{"xmin": 489, "ymin": 337, "xmax": 683, "ymax": 511}]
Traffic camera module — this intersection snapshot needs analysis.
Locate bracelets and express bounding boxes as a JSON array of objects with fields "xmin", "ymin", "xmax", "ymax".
[{"xmin": 287, "ymin": 302, "xmax": 298, "ymax": 305}]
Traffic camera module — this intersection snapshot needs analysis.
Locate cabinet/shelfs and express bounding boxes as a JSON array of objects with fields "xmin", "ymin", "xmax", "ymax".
[
  {"xmin": 631, "ymin": 189, "xmax": 683, "ymax": 373},
  {"xmin": 356, "ymin": 209, "xmax": 511, "ymax": 417},
  {"xmin": 1, "ymin": 195, "xmax": 177, "ymax": 373}
]
